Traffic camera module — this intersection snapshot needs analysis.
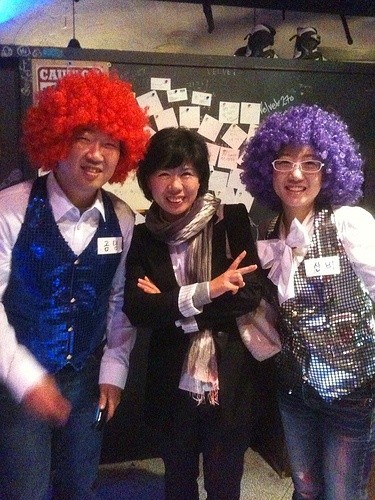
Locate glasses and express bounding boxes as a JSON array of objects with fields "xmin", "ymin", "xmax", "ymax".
[{"xmin": 272, "ymin": 157, "xmax": 325, "ymax": 173}]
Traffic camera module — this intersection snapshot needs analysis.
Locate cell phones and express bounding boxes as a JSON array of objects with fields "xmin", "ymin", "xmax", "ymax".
[{"xmin": 92, "ymin": 400, "xmax": 109, "ymax": 431}]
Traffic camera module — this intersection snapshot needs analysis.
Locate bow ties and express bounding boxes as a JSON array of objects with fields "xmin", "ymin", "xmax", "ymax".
[{"xmin": 256, "ymin": 217, "xmax": 311, "ymax": 307}]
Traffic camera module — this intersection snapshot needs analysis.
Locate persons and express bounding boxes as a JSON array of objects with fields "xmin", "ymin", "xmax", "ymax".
[
  {"xmin": 124, "ymin": 128, "xmax": 280, "ymax": 500},
  {"xmin": 0, "ymin": 69, "xmax": 149, "ymax": 500},
  {"xmin": 240, "ymin": 105, "xmax": 375, "ymax": 500}
]
